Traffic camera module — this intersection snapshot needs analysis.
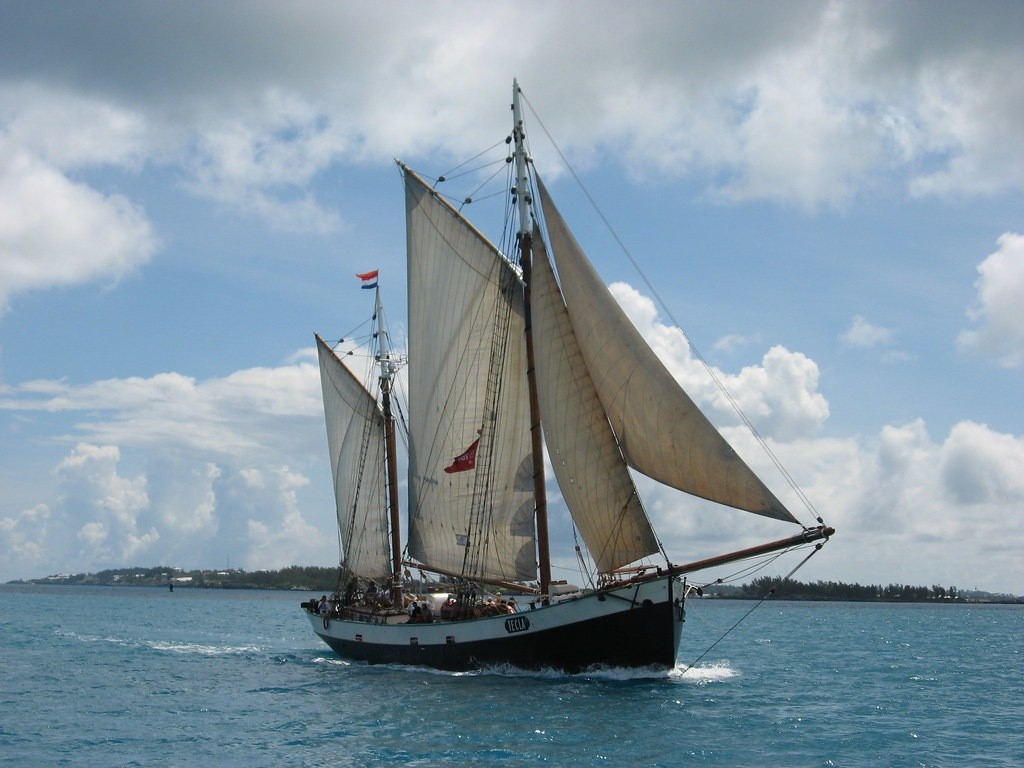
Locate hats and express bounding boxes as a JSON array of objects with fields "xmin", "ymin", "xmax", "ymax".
[{"xmin": 405, "ymin": 588, "xmax": 410, "ymax": 592}]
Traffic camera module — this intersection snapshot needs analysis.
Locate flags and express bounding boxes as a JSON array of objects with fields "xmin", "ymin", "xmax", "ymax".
[
  {"xmin": 444, "ymin": 436, "xmax": 480, "ymax": 474},
  {"xmin": 355, "ymin": 270, "xmax": 378, "ymax": 289}
]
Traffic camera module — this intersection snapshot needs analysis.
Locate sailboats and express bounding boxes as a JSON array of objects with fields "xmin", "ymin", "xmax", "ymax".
[{"xmin": 302, "ymin": 73, "xmax": 834, "ymax": 676}]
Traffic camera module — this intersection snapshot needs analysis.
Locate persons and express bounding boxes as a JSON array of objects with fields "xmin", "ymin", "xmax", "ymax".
[
  {"xmin": 318, "ymin": 595, "xmax": 331, "ymax": 615},
  {"xmin": 365, "ymin": 581, "xmax": 517, "ymax": 624}
]
